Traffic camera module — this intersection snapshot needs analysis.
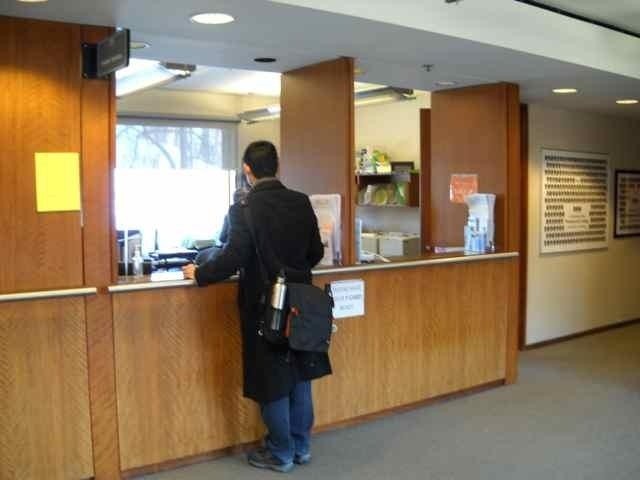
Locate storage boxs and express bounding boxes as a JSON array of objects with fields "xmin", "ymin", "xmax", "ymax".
[{"xmin": 359, "ymin": 232, "xmax": 420, "ymax": 258}]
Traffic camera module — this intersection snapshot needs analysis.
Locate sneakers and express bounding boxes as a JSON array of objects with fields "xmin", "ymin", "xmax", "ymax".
[{"xmin": 250, "ymin": 433, "xmax": 310, "ymax": 472}]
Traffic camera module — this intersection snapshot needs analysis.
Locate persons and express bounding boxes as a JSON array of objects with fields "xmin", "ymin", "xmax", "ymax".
[
  {"xmin": 181, "ymin": 140, "xmax": 332, "ymax": 472},
  {"xmin": 219, "ymin": 189, "xmax": 249, "ymax": 242}
]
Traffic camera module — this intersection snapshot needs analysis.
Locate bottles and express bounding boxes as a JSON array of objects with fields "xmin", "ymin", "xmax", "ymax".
[
  {"xmin": 130, "ymin": 247, "xmax": 144, "ymax": 279},
  {"xmin": 265, "ymin": 276, "xmax": 288, "ymax": 332},
  {"xmin": 464, "ymin": 217, "xmax": 487, "ymax": 255}
]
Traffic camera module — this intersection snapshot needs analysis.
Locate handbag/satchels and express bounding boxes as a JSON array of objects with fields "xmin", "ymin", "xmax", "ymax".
[{"xmin": 255, "ymin": 283, "xmax": 333, "ymax": 354}]
